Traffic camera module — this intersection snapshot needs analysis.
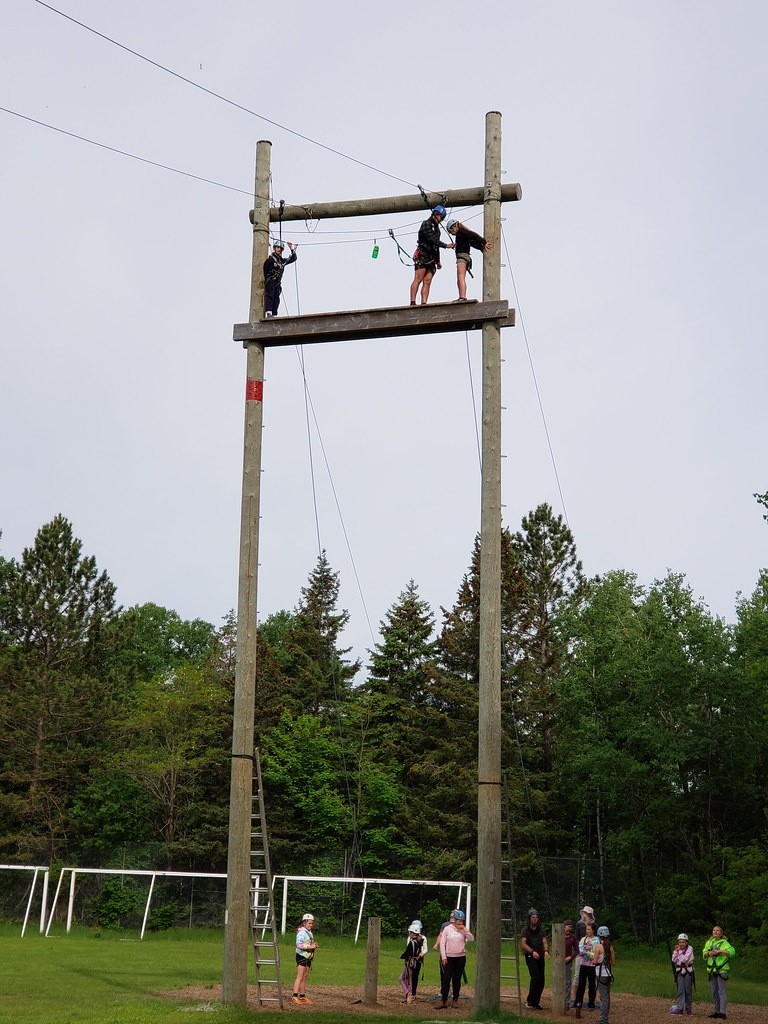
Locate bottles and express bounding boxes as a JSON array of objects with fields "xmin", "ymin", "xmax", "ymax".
[{"xmin": 372, "ymin": 246, "xmax": 379, "ymax": 258}]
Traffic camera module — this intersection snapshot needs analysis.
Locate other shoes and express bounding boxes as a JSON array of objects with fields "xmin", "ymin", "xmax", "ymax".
[
  {"xmin": 671, "ymin": 1009, "xmax": 684, "ymax": 1016},
  {"xmin": 598, "ymin": 1018, "xmax": 608, "ymax": 1024},
  {"xmin": 525, "ymin": 1002, "xmax": 544, "ymax": 1011},
  {"xmin": 583, "ymin": 1003, "xmax": 595, "ymax": 1010},
  {"xmin": 570, "ymin": 1003, "xmax": 582, "ymax": 1009},
  {"xmin": 452, "ymin": 297, "xmax": 467, "ymax": 302},
  {"xmin": 434, "ymin": 1003, "xmax": 448, "ymax": 1010},
  {"xmin": 412, "ymin": 996, "xmax": 416, "ymax": 1000},
  {"xmin": 708, "ymin": 1012, "xmax": 727, "ymax": 1021},
  {"xmin": 406, "ymin": 991, "xmax": 412, "ymax": 1004},
  {"xmin": 685, "ymin": 1007, "xmax": 693, "ymax": 1016},
  {"xmin": 452, "ymin": 1000, "xmax": 459, "ymax": 1008}
]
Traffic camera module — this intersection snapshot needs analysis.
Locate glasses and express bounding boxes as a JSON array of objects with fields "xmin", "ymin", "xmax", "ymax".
[{"xmin": 438, "ymin": 215, "xmax": 443, "ymax": 221}]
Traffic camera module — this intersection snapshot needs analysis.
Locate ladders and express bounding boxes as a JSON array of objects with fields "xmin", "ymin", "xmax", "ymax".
[
  {"xmin": 249, "ymin": 748, "xmax": 284, "ymax": 1007},
  {"xmin": 501, "ymin": 773, "xmax": 522, "ymax": 1015}
]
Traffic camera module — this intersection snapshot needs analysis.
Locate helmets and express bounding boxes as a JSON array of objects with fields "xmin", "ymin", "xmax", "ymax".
[
  {"xmin": 412, "ymin": 920, "xmax": 422, "ymax": 928},
  {"xmin": 678, "ymin": 933, "xmax": 689, "ymax": 940},
  {"xmin": 454, "ymin": 911, "xmax": 465, "ymax": 920},
  {"xmin": 433, "ymin": 205, "xmax": 446, "ymax": 217},
  {"xmin": 302, "ymin": 914, "xmax": 314, "ymax": 921},
  {"xmin": 597, "ymin": 925, "xmax": 610, "ymax": 937},
  {"xmin": 447, "ymin": 219, "xmax": 458, "ymax": 231},
  {"xmin": 273, "ymin": 240, "xmax": 284, "ymax": 249},
  {"xmin": 408, "ymin": 924, "xmax": 420, "ymax": 933}
]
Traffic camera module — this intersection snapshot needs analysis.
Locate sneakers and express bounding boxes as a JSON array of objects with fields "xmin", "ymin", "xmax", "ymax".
[
  {"xmin": 299, "ymin": 997, "xmax": 313, "ymax": 1005},
  {"xmin": 292, "ymin": 996, "xmax": 303, "ymax": 1005}
]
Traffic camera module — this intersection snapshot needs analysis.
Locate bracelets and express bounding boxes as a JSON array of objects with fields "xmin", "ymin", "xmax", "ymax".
[
  {"xmin": 531, "ymin": 950, "xmax": 534, "ymax": 954},
  {"xmin": 544, "ymin": 950, "xmax": 549, "ymax": 954}
]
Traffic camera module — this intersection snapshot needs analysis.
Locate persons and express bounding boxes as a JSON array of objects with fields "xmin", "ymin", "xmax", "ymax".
[
  {"xmin": 433, "ymin": 911, "xmax": 474, "ymax": 1009},
  {"xmin": 410, "ymin": 205, "xmax": 455, "ymax": 306},
  {"xmin": 669, "ymin": 933, "xmax": 695, "ymax": 1017},
  {"xmin": 702, "ymin": 927, "xmax": 736, "ymax": 1020},
  {"xmin": 571, "ymin": 906, "xmax": 600, "ymax": 1006},
  {"xmin": 291, "ymin": 914, "xmax": 319, "ymax": 1004},
  {"xmin": 564, "ymin": 920, "xmax": 580, "ymax": 1009},
  {"xmin": 446, "ymin": 219, "xmax": 493, "ymax": 302},
  {"xmin": 591, "ymin": 926, "xmax": 615, "ymax": 1024},
  {"xmin": 433, "ymin": 909, "xmax": 459, "ymax": 1001},
  {"xmin": 398, "ymin": 920, "xmax": 428, "ymax": 1003},
  {"xmin": 574, "ymin": 924, "xmax": 600, "ymax": 1011},
  {"xmin": 520, "ymin": 908, "xmax": 551, "ymax": 1010},
  {"xmin": 263, "ymin": 241, "xmax": 297, "ymax": 319}
]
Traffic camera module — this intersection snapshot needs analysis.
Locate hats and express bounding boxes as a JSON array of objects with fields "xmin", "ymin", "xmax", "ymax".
[
  {"xmin": 580, "ymin": 906, "xmax": 594, "ymax": 918},
  {"xmin": 527, "ymin": 908, "xmax": 538, "ymax": 915}
]
